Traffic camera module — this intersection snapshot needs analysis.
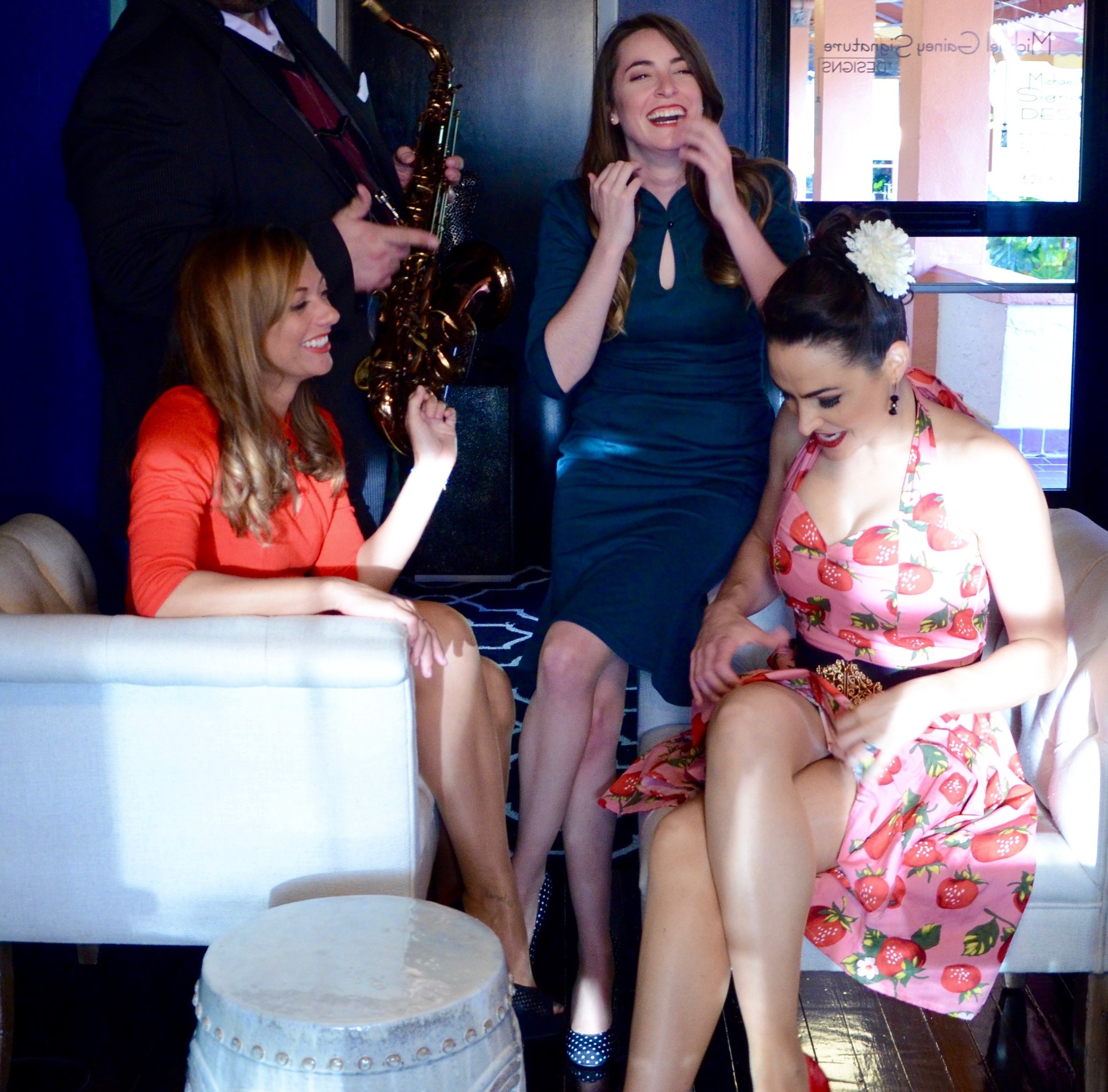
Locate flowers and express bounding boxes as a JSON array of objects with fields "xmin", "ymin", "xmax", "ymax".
[{"xmin": 843, "ymin": 217, "xmax": 918, "ymax": 298}]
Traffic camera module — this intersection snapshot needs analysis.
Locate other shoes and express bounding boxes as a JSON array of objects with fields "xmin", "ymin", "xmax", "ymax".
[
  {"xmin": 806, "ymin": 1054, "xmax": 830, "ymax": 1092},
  {"xmin": 456, "ymin": 890, "xmax": 562, "ymax": 1032}
]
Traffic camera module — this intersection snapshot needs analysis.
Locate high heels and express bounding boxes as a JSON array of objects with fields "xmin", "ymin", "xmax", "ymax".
[
  {"xmin": 565, "ymin": 926, "xmax": 618, "ymax": 1067},
  {"xmin": 524, "ymin": 869, "xmax": 553, "ymax": 958}
]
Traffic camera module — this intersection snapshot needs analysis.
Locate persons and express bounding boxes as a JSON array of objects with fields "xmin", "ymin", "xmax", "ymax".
[
  {"xmin": 61, "ymin": 1, "xmax": 464, "ymax": 615},
  {"xmin": 597, "ymin": 212, "xmax": 1067, "ymax": 1091},
  {"xmin": 512, "ymin": 14, "xmax": 810, "ymax": 1068},
  {"xmin": 127, "ymin": 234, "xmax": 569, "ymax": 1040}
]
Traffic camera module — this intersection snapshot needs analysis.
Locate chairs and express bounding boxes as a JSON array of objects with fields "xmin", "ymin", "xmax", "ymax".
[
  {"xmin": 0, "ymin": 512, "xmax": 438, "ymax": 946},
  {"xmin": 636, "ymin": 504, "xmax": 1108, "ymax": 1060}
]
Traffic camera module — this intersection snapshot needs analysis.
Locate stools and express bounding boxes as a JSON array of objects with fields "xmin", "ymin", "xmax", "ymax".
[{"xmin": 182, "ymin": 894, "xmax": 526, "ymax": 1092}]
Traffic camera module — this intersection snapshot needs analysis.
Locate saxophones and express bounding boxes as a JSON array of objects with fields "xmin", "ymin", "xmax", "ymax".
[{"xmin": 353, "ymin": 0, "xmax": 521, "ymax": 463}]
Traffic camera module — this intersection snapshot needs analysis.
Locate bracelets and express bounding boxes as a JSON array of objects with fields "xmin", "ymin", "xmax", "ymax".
[{"xmin": 410, "ymin": 468, "xmax": 447, "ymax": 490}]
